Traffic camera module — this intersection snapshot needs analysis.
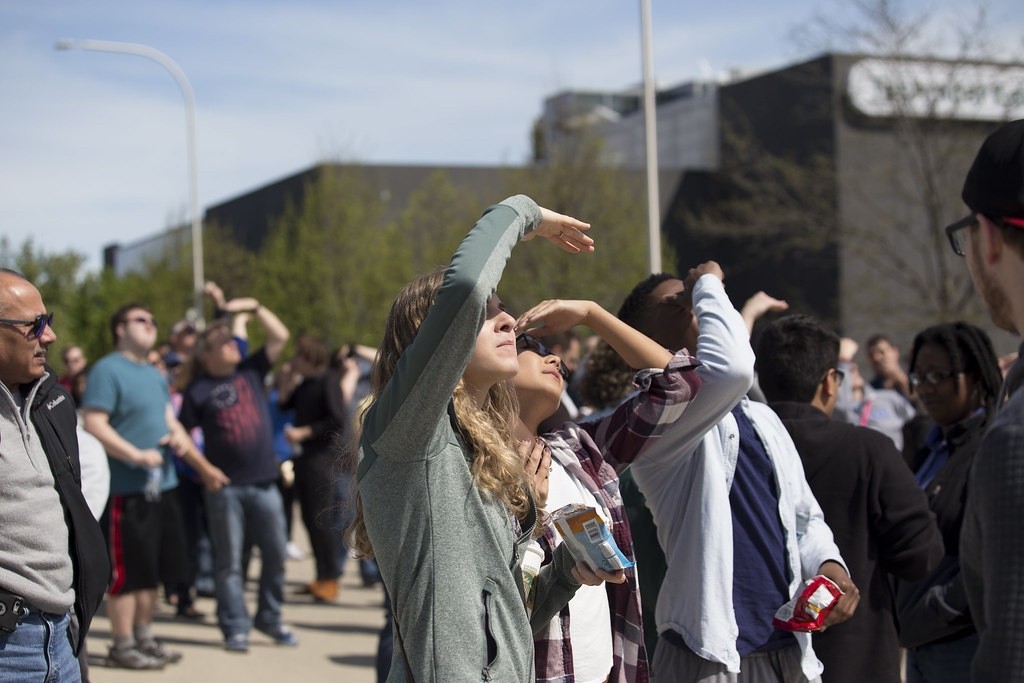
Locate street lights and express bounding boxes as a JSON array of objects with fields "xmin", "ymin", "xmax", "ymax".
[{"xmin": 53, "ymin": 37, "xmax": 207, "ymax": 321}]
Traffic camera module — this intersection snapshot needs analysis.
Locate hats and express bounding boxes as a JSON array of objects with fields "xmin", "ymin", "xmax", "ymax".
[{"xmin": 962, "ymin": 119, "xmax": 1024, "ymax": 220}]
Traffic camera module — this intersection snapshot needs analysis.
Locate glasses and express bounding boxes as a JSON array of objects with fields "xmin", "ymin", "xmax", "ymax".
[
  {"xmin": 126, "ymin": 317, "xmax": 156, "ymax": 327},
  {"xmin": 517, "ymin": 333, "xmax": 570, "ymax": 379},
  {"xmin": 820, "ymin": 369, "xmax": 845, "ymax": 387},
  {"xmin": 910, "ymin": 366, "xmax": 968, "ymax": 385},
  {"xmin": 0, "ymin": 312, "xmax": 53, "ymax": 337},
  {"xmin": 945, "ymin": 212, "xmax": 1023, "ymax": 258}
]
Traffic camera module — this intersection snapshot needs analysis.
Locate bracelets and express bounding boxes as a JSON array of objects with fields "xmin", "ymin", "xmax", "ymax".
[
  {"xmin": 346, "ymin": 343, "xmax": 356, "ymax": 358},
  {"xmin": 250, "ymin": 302, "xmax": 262, "ymax": 314}
]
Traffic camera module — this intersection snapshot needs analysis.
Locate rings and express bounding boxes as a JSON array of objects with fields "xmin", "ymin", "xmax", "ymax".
[{"xmin": 558, "ymin": 232, "xmax": 564, "ymax": 239}]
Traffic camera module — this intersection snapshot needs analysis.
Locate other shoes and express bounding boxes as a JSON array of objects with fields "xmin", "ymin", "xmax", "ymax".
[
  {"xmin": 225, "ymin": 632, "xmax": 249, "ymax": 650},
  {"xmin": 306, "ymin": 578, "xmax": 338, "ymax": 602},
  {"xmin": 135, "ymin": 637, "xmax": 182, "ymax": 662},
  {"xmin": 107, "ymin": 643, "xmax": 164, "ymax": 668},
  {"xmin": 175, "ymin": 604, "xmax": 203, "ymax": 622},
  {"xmin": 256, "ymin": 622, "xmax": 297, "ymax": 645}
]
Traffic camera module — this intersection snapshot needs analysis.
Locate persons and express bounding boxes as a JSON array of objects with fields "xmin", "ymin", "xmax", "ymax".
[
  {"xmin": 0, "ymin": 268, "xmax": 110, "ymax": 683},
  {"xmin": 498, "ymin": 119, "xmax": 1024, "ymax": 683},
  {"xmin": 344, "ymin": 195, "xmax": 627, "ymax": 683},
  {"xmin": 59, "ymin": 282, "xmax": 383, "ymax": 671}
]
